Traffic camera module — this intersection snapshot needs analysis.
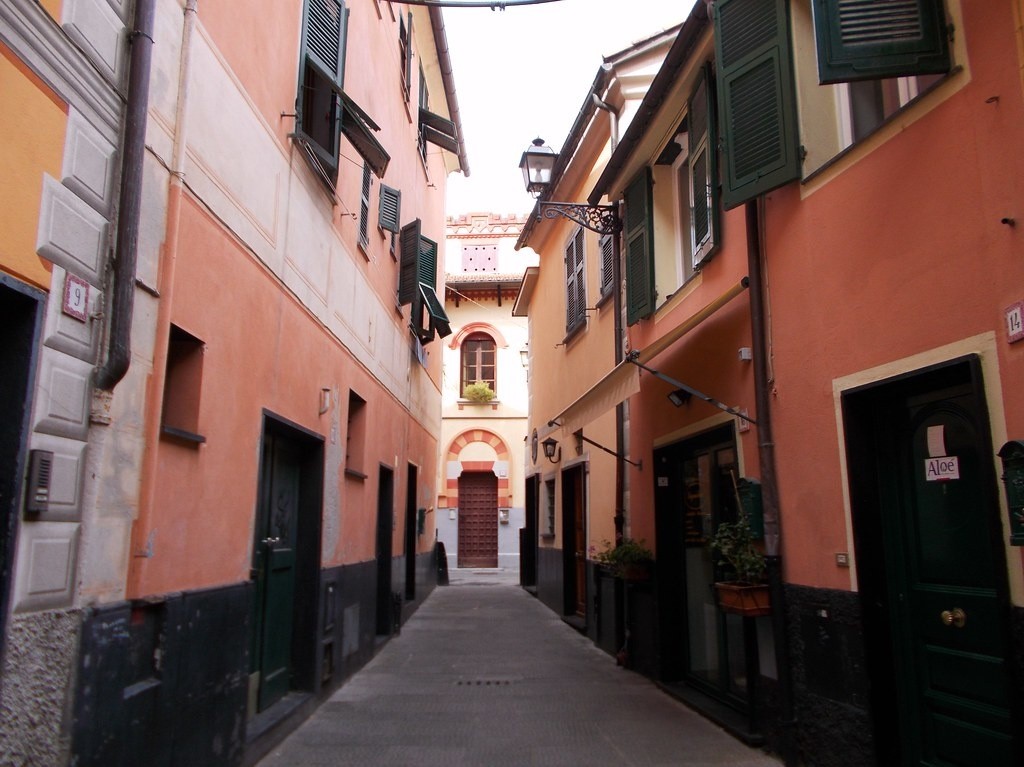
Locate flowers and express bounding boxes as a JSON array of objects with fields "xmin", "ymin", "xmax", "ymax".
[
  {"xmin": 700, "ymin": 511, "xmax": 766, "ymax": 584},
  {"xmin": 591, "ymin": 532, "xmax": 653, "ymax": 580}
]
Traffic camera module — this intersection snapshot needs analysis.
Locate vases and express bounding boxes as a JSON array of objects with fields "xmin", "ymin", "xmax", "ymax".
[
  {"xmin": 715, "ymin": 580, "xmax": 775, "ymax": 618},
  {"xmin": 616, "ymin": 561, "xmax": 648, "ymax": 580}
]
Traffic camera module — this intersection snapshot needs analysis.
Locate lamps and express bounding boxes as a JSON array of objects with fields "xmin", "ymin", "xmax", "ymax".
[
  {"xmin": 667, "ymin": 385, "xmax": 692, "ymax": 408},
  {"xmin": 518, "ymin": 135, "xmax": 624, "ymax": 236},
  {"xmin": 519, "ymin": 340, "xmax": 528, "ymax": 383},
  {"xmin": 541, "ymin": 437, "xmax": 561, "ymax": 464}
]
